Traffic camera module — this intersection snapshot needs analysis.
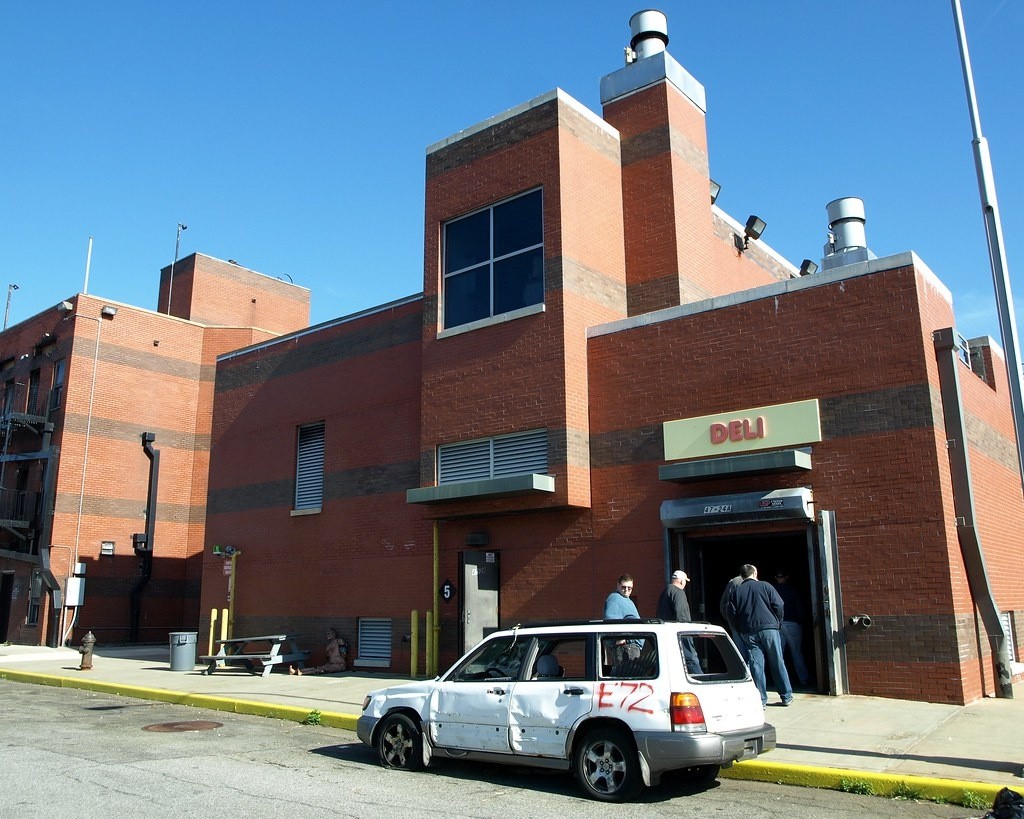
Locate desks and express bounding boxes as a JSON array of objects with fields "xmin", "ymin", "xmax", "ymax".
[{"xmin": 202, "ymin": 633, "xmax": 305, "ymax": 677}]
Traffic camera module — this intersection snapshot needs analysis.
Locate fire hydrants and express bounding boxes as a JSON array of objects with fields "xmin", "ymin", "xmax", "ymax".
[{"xmin": 77, "ymin": 630, "xmax": 97, "ymax": 671}]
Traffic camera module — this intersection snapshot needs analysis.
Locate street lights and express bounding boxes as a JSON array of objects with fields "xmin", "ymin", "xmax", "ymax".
[
  {"xmin": 167, "ymin": 220, "xmax": 188, "ymax": 316},
  {"xmin": 2, "ymin": 283, "xmax": 19, "ymax": 332}
]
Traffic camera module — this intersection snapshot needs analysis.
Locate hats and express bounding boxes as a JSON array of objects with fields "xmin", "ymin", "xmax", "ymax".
[
  {"xmin": 672, "ymin": 570, "xmax": 690, "ymax": 581},
  {"xmin": 775, "ymin": 569, "xmax": 785, "ymax": 578}
]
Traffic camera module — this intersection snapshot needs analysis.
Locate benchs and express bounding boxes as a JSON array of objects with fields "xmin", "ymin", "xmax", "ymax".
[{"xmin": 199, "ymin": 650, "xmax": 312, "ymax": 659}]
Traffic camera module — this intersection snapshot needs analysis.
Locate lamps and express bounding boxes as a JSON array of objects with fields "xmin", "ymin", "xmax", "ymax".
[
  {"xmin": 57, "ymin": 301, "xmax": 73, "ymax": 311},
  {"xmin": 710, "ymin": 179, "xmax": 721, "ymax": 205},
  {"xmin": 102, "ymin": 305, "xmax": 117, "ymax": 314},
  {"xmin": 800, "ymin": 260, "xmax": 818, "ymax": 276},
  {"xmin": 735, "ymin": 215, "xmax": 766, "ymax": 253}
]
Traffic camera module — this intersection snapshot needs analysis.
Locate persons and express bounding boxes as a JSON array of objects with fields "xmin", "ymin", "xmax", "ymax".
[
  {"xmin": 773, "ymin": 569, "xmax": 812, "ymax": 689},
  {"xmin": 729, "ymin": 564, "xmax": 794, "ymax": 705},
  {"xmin": 720, "ymin": 573, "xmax": 748, "ymax": 664},
  {"xmin": 603, "ymin": 574, "xmax": 644, "ymax": 664},
  {"xmin": 657, "ymin": 569, "xmax": 703, "ymax": 674},
  {"xmin": 289, "ymin": 629, "xmax": 347, "ymax": 675}
]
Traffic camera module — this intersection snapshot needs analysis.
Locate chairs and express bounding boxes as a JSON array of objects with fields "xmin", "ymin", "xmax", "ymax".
[{"xmin": 532, "ymin": 655, "xmax": 560, "ymax": 681}]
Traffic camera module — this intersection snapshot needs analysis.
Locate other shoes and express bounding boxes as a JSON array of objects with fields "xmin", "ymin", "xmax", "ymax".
[
  {"xmin": 763, "ymin": 703, "xmax": 766, "ymax": 710},
  {"xmin": 791, "ymin": 683, "xmax": 794, "ymax": 687},
  {"xmin": 801, "ymin": 683, "xmax": 813, "ymax": 691},
  {"xmin": 783, "ymin": 696, "xmax": 794, "ymax": 706}
]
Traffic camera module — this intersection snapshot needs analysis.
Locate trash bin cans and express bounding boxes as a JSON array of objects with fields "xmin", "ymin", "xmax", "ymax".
[{"xmin": 169, "ymin": 632, "xmax": 198, "ymax": 672}]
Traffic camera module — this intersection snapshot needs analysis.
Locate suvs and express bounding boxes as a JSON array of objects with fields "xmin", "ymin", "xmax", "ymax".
[{"xmin": 355, "ymin": 616, "xmax": 776, "ymax": 804}]
